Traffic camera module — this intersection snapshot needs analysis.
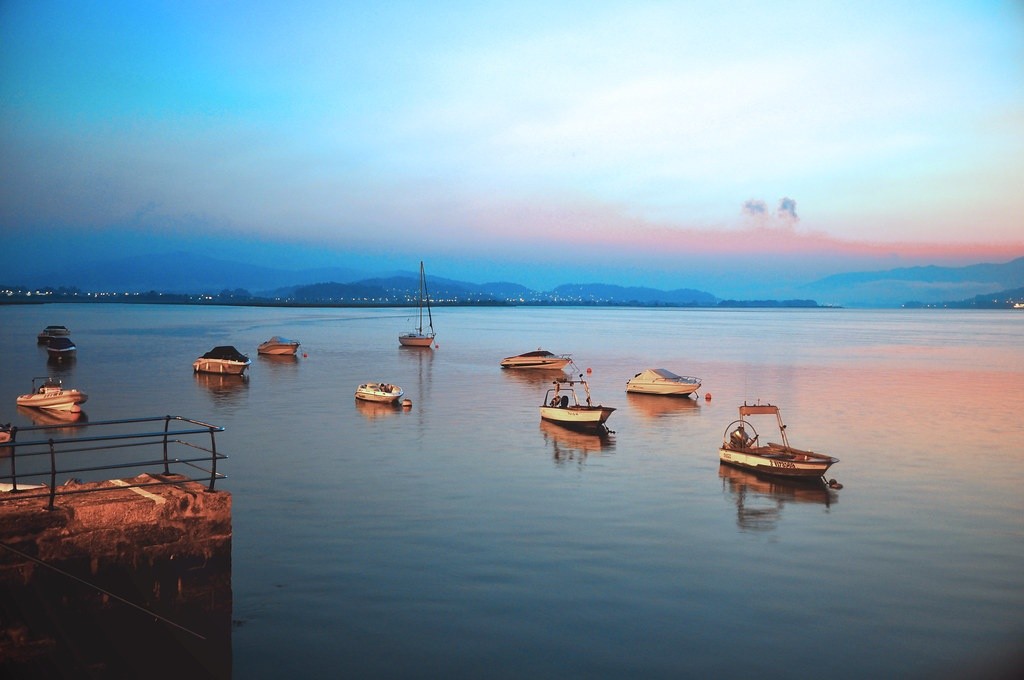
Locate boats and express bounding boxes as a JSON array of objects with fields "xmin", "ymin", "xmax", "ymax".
[
  {"xmin": 192, "ymin": 346, "xmax": 252, "ymax": 374},
  {"xmin": 537, "ymin": 377, "xmax": 616, "ymax": 428},
  {"xmin": 0, "ymin": 421, "xmax": 15, "ymax": 442},
  {"xmin": 47, "ymin": 337, "xmax": 76, "ymax": 353},
  {"xmin": 44, "ymin": 325, "xmax": 71, "ymax": 334},
  {"xmin": 37, "ymin": 333, "xmax": 55, "ymax": 343},
  {"xmin": 499, "ymin": 349, "xmax": 572, "ymax": 369},
  {"xmin": 17, "ymin": 378, "xmax": 88, "ymax": 412},
  {"xmin": 257, "ymin": 335, "xmax": 301, "ymax": 355},
  {"xmin": 355, "ymin": 398, "xmax": 403, "ymax": 420},
  {"xmin": 258, "ymin": 352, "xmax": 298, "ymax": 366},
  {"xmin": 354, "ymin": 382, "xmax": 404, "ymax": 404},
  {"xmin": 626, "ymin": 369, "xmax": 703, "ymax": 397},
  {"xmin": 719, "ymin": 404, "xmax": 843, "ymax": 490},
  {"xmin": 539, "ymin": 417, "xmax": 610, "ymax": 464},
  {"xmin": 718, "ymin": 461, "xmax": 843, "ymax": 533},
  {"xmin": 17, "ymin": 404, "xmax": 83, "ymax": 431},
  {"xmin": 192, "ymin": 370, "xmax": 250, "ymax": 396},
  {"xmin": 626, "ymin": 391, "xmax": 701, "ymax": 423}
]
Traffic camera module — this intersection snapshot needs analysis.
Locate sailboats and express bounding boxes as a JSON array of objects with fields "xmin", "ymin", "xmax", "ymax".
[
  {"xmin": 399, "ymin": 260, "xmax": 437, "ymax": 348},
  {"xmin": 398, "ymin": 345, "xmax": 433, "ymax": 447}
]
